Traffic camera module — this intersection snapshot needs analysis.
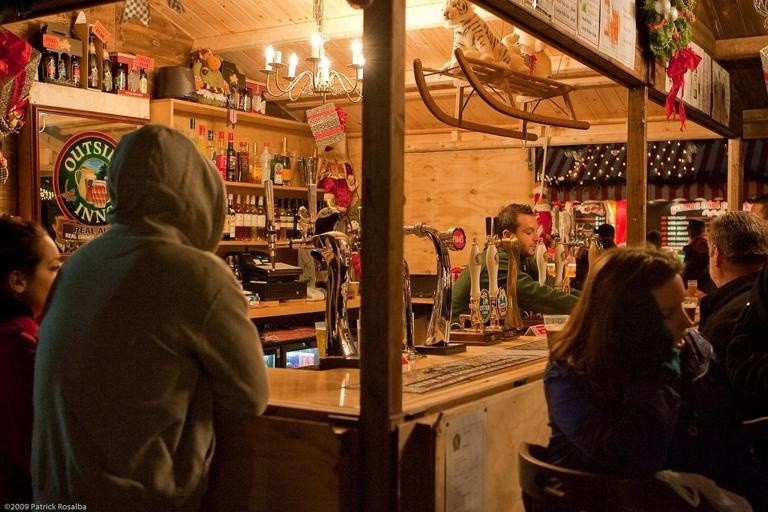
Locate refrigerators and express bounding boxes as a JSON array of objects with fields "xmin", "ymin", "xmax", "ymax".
[{"xmin": 260, "ymin": 325, "xmax": 320, "ymax": 368}]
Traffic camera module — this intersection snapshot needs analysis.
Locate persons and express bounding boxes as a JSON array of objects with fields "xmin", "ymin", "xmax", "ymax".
[
  {"xmin": 683, "ymin": 219, "xmax": 708, "ymax": 291},
  {"xmin": 1, "ymin": 214, "xmax": 62, "ymax": 512},
  {"xmin": 451, "ymin": 204, "xmax": 582, "ymax": 330},
  {"xmin": 541, "ymin": 245, "xmax": 752, "ymax": 512},
  {"xmin": 31, "ymin": 121, "xmax": 271, "ymax": 508},
  {"xmin": 697, "ymin": 210, "xmax": 768, "ymax": 511},
  {"xmin": 440, "ymin": 0, "xmax": 513, "ymax": 79},
  {"xmin": 595, "ymin": 224, "xmax": 616, "ymax": 250},
  {"xmin": 646, "ymin": 229, "xmax": 662, "ymax": 250}
]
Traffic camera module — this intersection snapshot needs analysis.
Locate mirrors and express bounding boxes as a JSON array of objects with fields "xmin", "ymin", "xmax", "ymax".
[{"xmin": 34, "ymin": 106, "xmax": 149, "ymax": 260}]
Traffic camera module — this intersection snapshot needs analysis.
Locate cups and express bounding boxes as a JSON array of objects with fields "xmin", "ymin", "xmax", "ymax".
[
  {"xmin": 683, "ymin": 304, "xmax": 695, "ymax": 322},
  {"xmin": 687, "ymin": 278, "xmax": 697, "ymax": 298},
  {"xmin": 542, "ymin": 314, "xmax": 572, "ymax": 351},
  {"xmin": 84, "ymin": 176, "xmax": 108, "ymax": 209}
]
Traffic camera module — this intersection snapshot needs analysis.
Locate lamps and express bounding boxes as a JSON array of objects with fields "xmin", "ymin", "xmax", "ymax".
[{"xmin": 260, "ymin": 0, "xmax": 364, "ymax": 104}]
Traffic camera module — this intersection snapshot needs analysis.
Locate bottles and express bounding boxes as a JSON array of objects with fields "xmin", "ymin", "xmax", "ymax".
[
  {"xmin": 241, "ymin": 83, "xmax": 268, "ymax": 114},
  {"xmin": 221, "ymin": 193, "xmax": 329, "ymax": 242},
  {"xmin": 267, "ymin": 354, "xmax": 315, "ymax": 367},
  {"xmin": 44, "ymin": 35, "xmax": 148, "ymax": 95},
  {"xmin": 187, "ymin": 117, "xmax": 291, "ymax": 186},
  {"xmin": 225, "ymin": 253, "xmax": 242, "ymax": 283}
]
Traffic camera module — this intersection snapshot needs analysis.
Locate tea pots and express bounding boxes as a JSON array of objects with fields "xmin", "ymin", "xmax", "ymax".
[{"xmin": 73, "ymin": 168, "xmax": 97, "ymax": 200}]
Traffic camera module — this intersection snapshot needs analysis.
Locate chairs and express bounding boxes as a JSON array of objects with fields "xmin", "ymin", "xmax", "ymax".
[{"xmin": 518, "ymin": 442, "xmax": 703, "ymax": 512}]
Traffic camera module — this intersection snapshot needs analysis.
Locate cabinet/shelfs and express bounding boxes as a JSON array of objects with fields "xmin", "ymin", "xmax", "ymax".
[{"xmin": 151, "ymin": 99, "xmax": 325, "ymax": 254}]
